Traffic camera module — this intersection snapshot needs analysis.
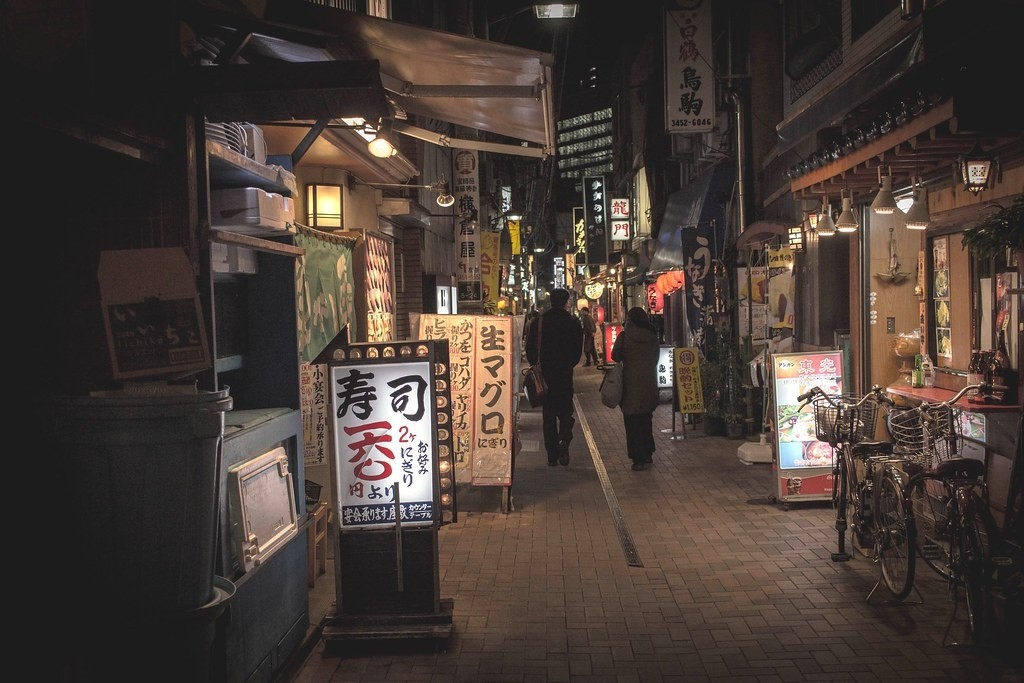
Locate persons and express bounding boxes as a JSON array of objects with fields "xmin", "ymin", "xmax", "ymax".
[
  {"xmin": 581, "ymin": 307, "xmax": 598, "ymax": 367},
  {"xmin": 611, "ymin": 307, "xmax": 660, "ymax": 470},
  {"xmin": 522, "ymin": 288, "xmax": 583, "ymax": 465}
]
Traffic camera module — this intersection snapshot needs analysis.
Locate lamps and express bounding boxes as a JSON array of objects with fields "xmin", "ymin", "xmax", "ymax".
[
  {"xmin": 835, "ymin": 175, "xmax": 859, "ymax": 232},
  {"xmin": 253, "ymin": 116, "xmax": 399, "ymax": 158},
  {"xmin": 303, "ymin": 183, "xmax": 343, "ymax": 232},
  {"xmin": 871, "ymin": 161, "xmax": 897, "ymax": 213},
  {"xmin": 488, "ymin": 208, "xmax": 523, "ymax": 227},
  {"xmin": 788, "ymin": 227, "xmax": 806, "ymax": 255},
  {"xmin": 955, "ymin": 132, "xmax": 1002, "ymax": 195},
  {"xmin": 904, "ymin": 146, "xmax": 932, "ymax": 229},
  {"xmin": 816, "ymin": 190, "xmax": 836, "ymax": 236},
  {"xmin": 350, "ymin": 174, "xmax": 455, "ymax": 207}
]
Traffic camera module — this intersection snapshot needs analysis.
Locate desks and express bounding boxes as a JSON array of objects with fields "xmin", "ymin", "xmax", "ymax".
[{"xmin": 886, "ymin": 385, "xmax": 1024, "ymax": 443}]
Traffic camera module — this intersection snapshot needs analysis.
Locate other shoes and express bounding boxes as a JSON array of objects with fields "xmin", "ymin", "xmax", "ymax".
[
  {"xmin": 558, "ymin": 440, "xmax": 569, "ymax": 466},
  {"xmin": 594, "ymin": 360, "xmax": 598, "ymax": 365},
  {"xmin": 583, "ymin": 362, "xmax": 591, "ymax": 367},
  {"xmin": 631, "ymin": 463, "xmax": 644, "ymax": 470},
  {"xmin": 548, "ymin": 459, "xmax": 557, "ymax": 466},
  {"xmin": 640, "ymin": 454, "xmax": 650, "ymax": 463}
]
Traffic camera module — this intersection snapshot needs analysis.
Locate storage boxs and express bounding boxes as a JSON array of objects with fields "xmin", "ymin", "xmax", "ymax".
[{"xmin": 210, "ymin": 186, "xmax": 296, "ymax": 238}]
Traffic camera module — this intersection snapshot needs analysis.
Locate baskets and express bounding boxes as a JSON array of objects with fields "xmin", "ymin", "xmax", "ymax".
[
  {"xmin": 814, "ymin": 397, "xmax": 879, "ymax": 443},
  {"xmin": 888, "ymin": 402, "xmax": 963, "ymax": 467}
]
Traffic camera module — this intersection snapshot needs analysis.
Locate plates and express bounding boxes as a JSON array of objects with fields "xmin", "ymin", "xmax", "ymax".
[
  {"xmin": 938, "ymin": 337, "xmax": 949, "ymax": 352},
  {"xmin": 805, "ymin": 380, "xmax": 842, "ymax": 408},
  {"xmin": 806, "ymin": 441, "xmax": 842, "ymax": 466},
  {"xmin": 936, "ymin": 271, "xmax": 948, "ymax": 296}
]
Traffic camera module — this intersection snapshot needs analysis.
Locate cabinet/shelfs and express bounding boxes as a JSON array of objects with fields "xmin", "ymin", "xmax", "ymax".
[{"xmin": 199, "ymin": 112, "xmax": 309, "ymax": 683}]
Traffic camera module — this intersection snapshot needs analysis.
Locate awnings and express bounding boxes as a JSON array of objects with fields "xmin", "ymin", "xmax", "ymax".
[
  {"xmin": 191, "ymin": 0, "xmax": 557, "ymax": 158},
  {"xmin": 646, "ymin": 165, "xmax": 766, "ymax": 274}
]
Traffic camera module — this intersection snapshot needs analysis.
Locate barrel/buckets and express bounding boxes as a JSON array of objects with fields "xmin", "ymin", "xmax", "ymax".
[
  {"xmin": 52, "ymin": 380, "xmax": 234, "ymax": 610},
  {"xmin": 70, "ymin": 574, "xmax": 236, "ymax": 683}
]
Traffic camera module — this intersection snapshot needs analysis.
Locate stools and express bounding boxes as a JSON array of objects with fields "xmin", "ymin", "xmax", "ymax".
[{"xmin": 304, "ymin": 500, "xmax": 327, "ymax": 587}]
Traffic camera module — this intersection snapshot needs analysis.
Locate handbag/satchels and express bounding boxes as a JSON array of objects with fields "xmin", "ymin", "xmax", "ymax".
[
  {"xmin": 522, "ymin": 363, "xmax": 549, "ymax": 408},
  {"xmin": 599, "ymin": 362, "xmax": 624, "ymax": 409}
]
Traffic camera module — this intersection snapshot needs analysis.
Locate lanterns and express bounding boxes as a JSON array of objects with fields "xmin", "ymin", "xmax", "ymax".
[
  {"xmin": 578, "ymin": 298, "xmax": 588, "ymax": 310},
  {"xmin": 957, "ymin": 149, "xmax": 1003, "ymax": 196},
  {"xmin": 656, "ymin": 271, "xmax": 684, "ymax": 294},
  {"xmin": 593, "ymin": 305, "xmax": 604, "ymax": 323},
  {"xmin": 648, "ymin": 283, "xmax": 664, "ymax": 312}
]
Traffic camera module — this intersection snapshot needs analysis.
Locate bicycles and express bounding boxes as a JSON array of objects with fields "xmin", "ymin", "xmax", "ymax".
[
  {"xmin": 796, "ymin": 384, "xmax": 925, "ymax": 607},
  {"xmin": 885, "ymin": 380, "xmax": 1014, "ymax": 650}
]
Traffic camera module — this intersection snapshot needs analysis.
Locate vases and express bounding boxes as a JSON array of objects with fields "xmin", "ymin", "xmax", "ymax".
[
  {"xmin": 888, "ymin": 406, "xmax": 923, "ymax": 453},
  {"xmin": 886, "ymin": 337, "xmax": 920, "ymax": 406}
]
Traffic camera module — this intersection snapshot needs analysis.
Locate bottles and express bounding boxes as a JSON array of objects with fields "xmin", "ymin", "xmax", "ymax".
[
  {"xmin": 912, "ymin": 355, "xmax": 924, "ymax": 388},
  {"xmin": 923, "ymin": 354, "xmax": 933, "ymax": 387},
  {"xmin": 967, "ymin": 329, "xmax": 1011, "ymax": 404}
]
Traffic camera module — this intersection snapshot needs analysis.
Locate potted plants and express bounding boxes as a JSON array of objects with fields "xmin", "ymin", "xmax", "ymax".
[
  {"xmin": 710, "ymin": 278, "xmax": 743, "ymax": 332},
  {"xmin": 693, "ymin": 333, "xmax": 745, "ymax": 440}
]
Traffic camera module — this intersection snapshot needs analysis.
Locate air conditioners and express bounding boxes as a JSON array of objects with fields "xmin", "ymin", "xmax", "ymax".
[
  {"xmin": 702, "ymin": 109, "xmax": 730, "ymax": 159},
  {"xmin": 671, "ymin": 133, "xmax": 698, "ymax": 156}
]
